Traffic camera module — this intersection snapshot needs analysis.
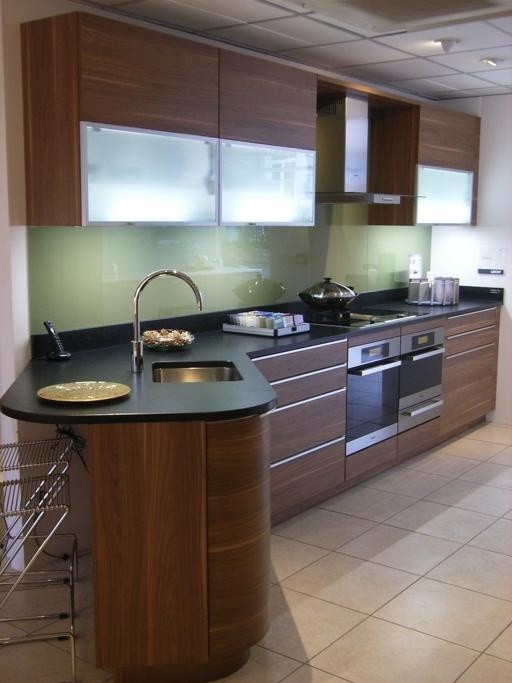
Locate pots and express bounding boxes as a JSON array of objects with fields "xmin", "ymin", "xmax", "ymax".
[{"xmin": 298, "ymin": 277, "xmax": 358, "ymax": 312}]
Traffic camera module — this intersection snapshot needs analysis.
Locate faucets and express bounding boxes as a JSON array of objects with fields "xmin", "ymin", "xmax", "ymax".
[{"xmin": 134, "ymin": 269, "xmax": 203, "ymax": 342}]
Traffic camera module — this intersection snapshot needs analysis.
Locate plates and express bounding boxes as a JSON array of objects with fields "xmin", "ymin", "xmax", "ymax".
[
  {"xmin": 37, "ymin": 380, "xmax": 132, "ymax": 404},
  {"xmin": 142, "ymin": 327, "xmax": 194, "ymax": 351}
]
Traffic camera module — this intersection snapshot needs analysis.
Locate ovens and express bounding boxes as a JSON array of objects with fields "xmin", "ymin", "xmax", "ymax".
[{"xmin": 343, "ymin": 326, "xmax": 448, "ymax": 457}]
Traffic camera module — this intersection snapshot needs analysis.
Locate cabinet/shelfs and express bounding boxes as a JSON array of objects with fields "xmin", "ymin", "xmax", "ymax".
[
  {"xmin": 441, "ymin": 307, "xmax": 500, "ymax": 442},
  {"xmin": 250, "ymin": 338, "xmax": 346, "ymax": 530},
  {"xmin": 21, "ymin": 11, "xmax": 317, "ymax": 226},
  {"xmin": 368, "ymin": 104, "xmax": 479, "ymax": 226}
]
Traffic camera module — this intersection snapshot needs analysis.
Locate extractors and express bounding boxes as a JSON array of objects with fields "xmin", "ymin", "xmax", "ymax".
[{"xmin": 316, "ymin": 90, "xmax": 427, "ymax": 205}]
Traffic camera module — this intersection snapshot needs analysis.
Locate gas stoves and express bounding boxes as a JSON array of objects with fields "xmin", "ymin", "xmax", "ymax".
[{"xmin": 277, "ymin": 276, "xmax": 430, "ymax": 330}]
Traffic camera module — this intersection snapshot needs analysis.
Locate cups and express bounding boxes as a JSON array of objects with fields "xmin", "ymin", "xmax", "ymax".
[{"xmin": 408, "ymin": 276, "xmax": 461, "ymax": 305}]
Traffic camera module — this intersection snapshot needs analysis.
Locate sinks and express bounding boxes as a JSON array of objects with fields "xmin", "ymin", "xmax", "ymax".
[{"xmin": 151, "ymin": 359, "xmax": 243, "ymax": 385}]
